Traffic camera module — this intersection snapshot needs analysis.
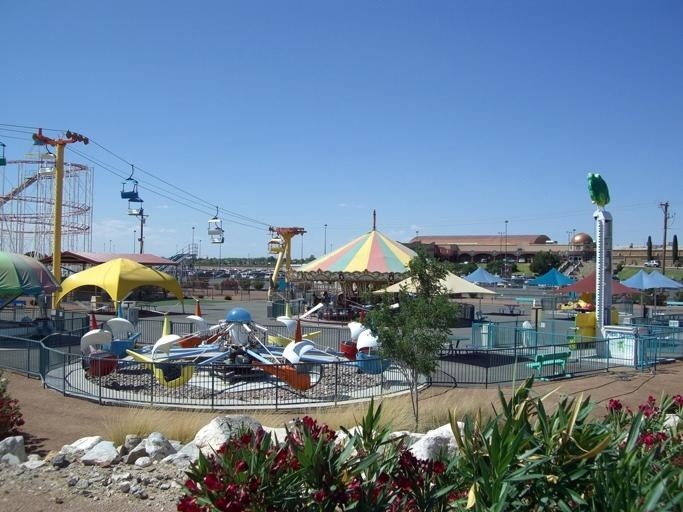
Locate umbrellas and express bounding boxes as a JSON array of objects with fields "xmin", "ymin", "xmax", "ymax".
[
  {"xmin": 372, "ymin": 266, "xmax": 497, "ymax": 296},
  {"xmin": 620, "ymin": 268, "xmax": 667, "ymax": 289},
  {"xmin": 0, "ymin": 250, "xmax": 65, "ymax": 297},
  {"xmin": 550, "ymin": 269, "xmax": 644, "ymax": 295},
  {"xmin": 648, "ymin": 270, "xmax": 683, "ymax": 289},
  {"xmin": 461, "ymin": 266, "xmax": 509, "ymax": 284},
  {"xmin": 528, "ymin": 268, "xmax": 577, "ymax": 287}
]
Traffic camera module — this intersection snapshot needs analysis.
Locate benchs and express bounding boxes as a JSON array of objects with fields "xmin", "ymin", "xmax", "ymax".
[{"xmin": 524, "ymin": 351, "xmax": 570, "ymax": 379}]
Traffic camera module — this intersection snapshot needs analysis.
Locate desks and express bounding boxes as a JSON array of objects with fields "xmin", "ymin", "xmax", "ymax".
[{"xmin": 449, "ymin": 336, "xmax": 471, "ymax": 353}]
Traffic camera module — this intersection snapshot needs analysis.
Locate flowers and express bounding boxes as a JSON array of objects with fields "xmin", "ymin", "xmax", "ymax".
[
  {"xmin": 604, "ymin": 389, "xmax": 683, "ymax": 472},
  {"xmin": 0, "ymin": 397, "xmax": 26, "ymax": 430},
  {"xmin": 168, "ymin": 413, "xmax": 446, "ymax": 510}
]
